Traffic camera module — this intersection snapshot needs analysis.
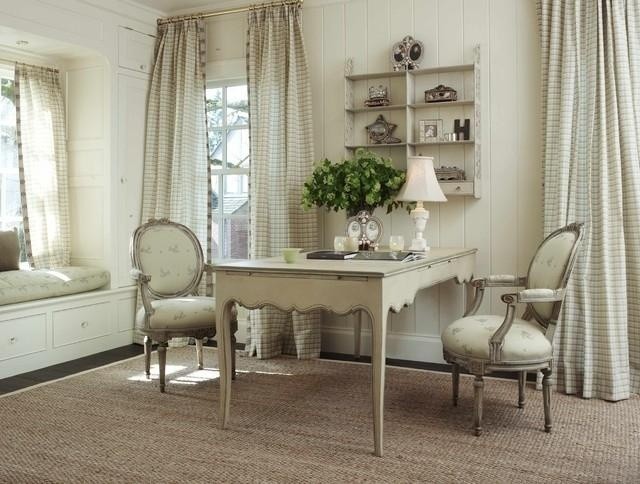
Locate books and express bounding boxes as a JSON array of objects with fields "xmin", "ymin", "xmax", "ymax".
[
  {"xmin": 306, "ymin": 251, "xmax": 360, "ymax": 260},
  {"xmin": 352, "ymin": 250, "xmax": 425, "ymax": 262}
]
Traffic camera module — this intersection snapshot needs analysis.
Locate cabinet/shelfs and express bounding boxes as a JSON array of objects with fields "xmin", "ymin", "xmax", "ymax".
[
  {"xmin": 0, "ymin": 265, "xmax": 137, "ymax": 379},
  {"xmin": 62, "ymin": 66, "xmax": 152, "ymax": 291},
  {"xmin": 117, "ymin": 24, "xmax": 163, "ymax": 73},
  {"xmin": 340, "ymin": 42, "xmax": 487, "ymax": 202}
]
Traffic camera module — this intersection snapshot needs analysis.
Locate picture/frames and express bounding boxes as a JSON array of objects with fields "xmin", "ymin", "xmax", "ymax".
[
  {"xmin": 417, "ymin": 118, "xmax": 444, "ymax": 144},
  {"xmin": 391, "ymin": 34, "xmax": 425, "ymax": 72}
]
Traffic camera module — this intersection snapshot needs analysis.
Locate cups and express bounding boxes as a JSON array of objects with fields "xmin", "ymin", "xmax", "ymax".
[
  {"xmin": 390, "ymin": 236, "xmax": 404, "ymax": 251},
  {"xmin": 443, "ymin": 132, "xmax": 456, "ymax": 141},
  {"xmin": 334, "ymin": 236, "xmax": 345, "ymax": 250},
  {"xmin": 343, "ymin": 238, "xmax": 359, "ymax": 251},
  {"xmin": 281, "ymin": 247, "xmax": 299, "ymax": 262}
]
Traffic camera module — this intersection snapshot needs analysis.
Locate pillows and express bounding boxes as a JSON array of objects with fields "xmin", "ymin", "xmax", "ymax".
[{"xmin": 0, "ymin": 228, "xmax": 20, "ymax": 273}]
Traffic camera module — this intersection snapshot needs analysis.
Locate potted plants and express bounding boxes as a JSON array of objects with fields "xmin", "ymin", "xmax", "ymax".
[{"xmin": 300, "ymin": 150, "xmax": 418, "ymax": 251}]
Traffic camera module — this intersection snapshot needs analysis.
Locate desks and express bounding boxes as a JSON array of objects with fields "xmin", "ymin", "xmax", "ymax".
[{"xmin": 208, "ymin": 246, "xmax": 479, "ymax": 460}]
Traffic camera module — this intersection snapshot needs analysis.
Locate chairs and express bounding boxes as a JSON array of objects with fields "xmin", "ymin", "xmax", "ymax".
[
  {"xmin": 129, "ymin": 215, "xmax": 240, "ymax": 394},
  {"xmin": 442, "ymin": 222, "xmax": 587, "ymax": 439}
]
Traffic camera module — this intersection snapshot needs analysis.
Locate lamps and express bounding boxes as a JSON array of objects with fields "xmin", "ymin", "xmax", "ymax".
[{"xmin": 395, "ymin": 155, "xmax": 449, "ymax": 254}]
